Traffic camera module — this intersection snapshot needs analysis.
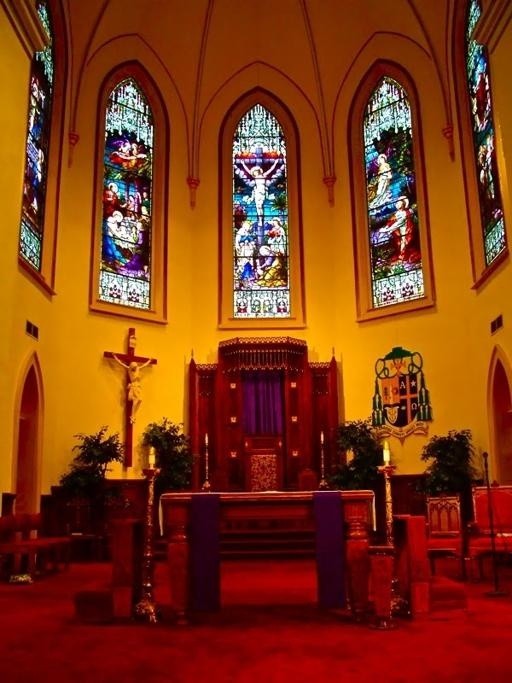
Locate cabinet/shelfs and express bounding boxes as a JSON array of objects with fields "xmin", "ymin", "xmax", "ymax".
[{"xmin": 425, "ymin": 495, "xmax": 462, "ymax": 538}]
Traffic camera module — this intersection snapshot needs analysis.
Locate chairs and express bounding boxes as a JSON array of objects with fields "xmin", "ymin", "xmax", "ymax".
[
  {"xmin": 464, "ymin": 485, "xmax": 512, "ymax": 581},
  {"xmin": 0, "ymin": 513, "xmax": 75, "ymax": 581}
]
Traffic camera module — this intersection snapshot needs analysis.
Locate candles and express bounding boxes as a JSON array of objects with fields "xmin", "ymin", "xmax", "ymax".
[
  {"xmin": 383, "ymin": 449, "xmax": 390, "ymax": 461},
  {"xmin": 320, "ymin": 431, "xmax": 324, "ymax": 444},
  {"xmin": 149, "ymin": 454, "xmax": 155, "ymax": 465},
  {"xmin": 205, "ymin": 433, "xmax": 208, "ymax": 445}
]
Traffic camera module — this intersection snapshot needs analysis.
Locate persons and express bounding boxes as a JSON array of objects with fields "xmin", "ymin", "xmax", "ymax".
[
  {"xmin": 112, "ymin": 351, "xmax": 152, "ymax": 423},
  {"xmin": 378, "ymin": 199, "xmax": 421, "ymax": 264},
  {"xmin": 111, "ymin": 137, "xmax": 148, "ymax": 161},
  {"xmin": 235, "ymin": 216, "xmax": 288, "ymax": 290},
  {"xmin": 100, "ymin": 182, "xmax": 152, "ymax": 275},
  {"xmin": 240, "ymin": 158, "xmax": 279, "ymax": 227},
  {"xmin": 367, "ymin": 155, "xmax": 392, "ymax": 209}
]
